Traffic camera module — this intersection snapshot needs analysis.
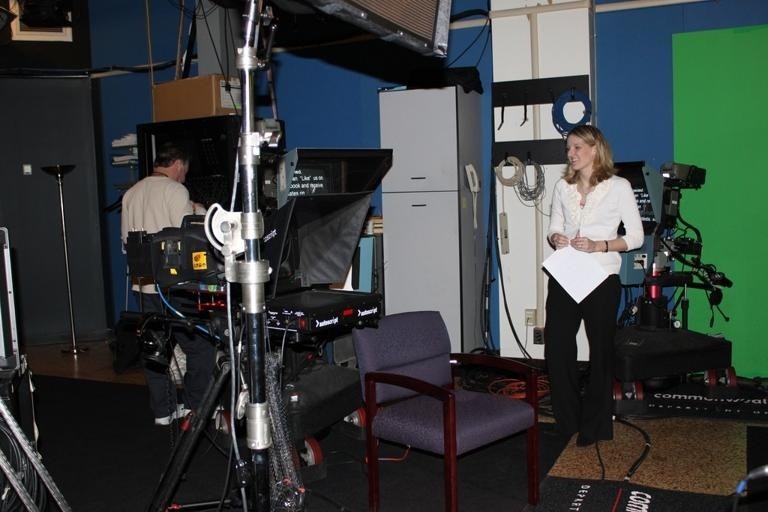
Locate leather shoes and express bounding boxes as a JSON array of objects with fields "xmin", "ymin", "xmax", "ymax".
[
  {"xmin": 543, "ymin": 429, "xmax": 564, "ymax": 440},
  {"xmin": 576, "ymin": 432, "xmax": 595, "ymax": 447}
]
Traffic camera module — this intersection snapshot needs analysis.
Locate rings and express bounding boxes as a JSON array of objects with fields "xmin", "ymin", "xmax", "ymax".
[
  {"xmin": 555, "ymin": 239, "xmax": 558, "ymax": 242},
  {"xmin": 581, "ymin": 244, "xmax": 582, "ymax": 248}
]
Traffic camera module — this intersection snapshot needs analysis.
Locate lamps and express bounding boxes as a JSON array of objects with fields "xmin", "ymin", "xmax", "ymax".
[{"xmin": 39, "ymin": 163, "xmax": 89, "ymax": 354}]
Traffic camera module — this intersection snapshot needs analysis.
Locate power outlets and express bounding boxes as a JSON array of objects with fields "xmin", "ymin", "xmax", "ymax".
[
  {"xmin": 524, "ymin": 307, "xmax": 537, "ymax": 326},
  {"xmin": 634, "ymin": 255, "xmax": 648, "ymax": 271}
]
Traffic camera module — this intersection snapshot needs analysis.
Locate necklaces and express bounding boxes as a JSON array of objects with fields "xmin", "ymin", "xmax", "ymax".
[{"xmin": 578, "ymin": 180, "xmax": 593, "ymax": 195}]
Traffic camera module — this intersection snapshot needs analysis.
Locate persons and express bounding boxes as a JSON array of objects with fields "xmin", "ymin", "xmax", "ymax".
[
  {"xmin": 540, "ymin": 124, "xmax": 646, "ymax": 450},
  {"xmin": 119, "ymin": 139, "xmax": 218, "ymax": 428}
]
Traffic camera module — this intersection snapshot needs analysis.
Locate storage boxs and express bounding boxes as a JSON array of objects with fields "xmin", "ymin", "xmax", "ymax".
[{"xmin": 151, "ymin": 74, "xmax": 248, "ymax": 122}]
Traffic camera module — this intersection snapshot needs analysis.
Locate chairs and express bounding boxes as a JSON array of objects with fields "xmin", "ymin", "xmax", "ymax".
[{"xmin": 352, "ymin": 310, "xmax": 539, "ymax": 512}]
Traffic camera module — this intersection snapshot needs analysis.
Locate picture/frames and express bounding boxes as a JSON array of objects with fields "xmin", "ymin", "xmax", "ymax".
[{"xmin": 9, "ymin": 0, "xmax": 72, "ymax": 42}]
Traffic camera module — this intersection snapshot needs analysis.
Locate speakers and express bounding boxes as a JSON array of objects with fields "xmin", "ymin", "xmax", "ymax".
[{"xmin": 0, "ymin": 368, "xmax": 40, "ymax": 512}]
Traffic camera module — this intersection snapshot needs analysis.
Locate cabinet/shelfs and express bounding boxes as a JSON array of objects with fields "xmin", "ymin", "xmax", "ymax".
[{"xmin": 380, "ymin": 85, "xmax": 484, "ymax": 354}]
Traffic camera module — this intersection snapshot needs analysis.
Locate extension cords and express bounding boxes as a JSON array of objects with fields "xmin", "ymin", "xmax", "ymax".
[{"xmin": 500, "ymin": 213, "xmax": 510, "ymax": 255}]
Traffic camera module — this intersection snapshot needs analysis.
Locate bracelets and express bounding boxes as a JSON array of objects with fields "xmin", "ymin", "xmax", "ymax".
[{"xmin": 601, "ymin": 239, "xmax": 608, "ymax": 253}]
